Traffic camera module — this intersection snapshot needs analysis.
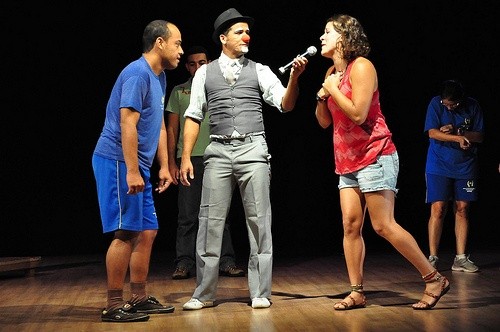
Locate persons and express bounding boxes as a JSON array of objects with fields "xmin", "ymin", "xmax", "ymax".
[
  {"xmin": 166, "ymin": 49, "xmax": 245, "ymax": 279},
  {"xmin": 316, "ymin": 15, "xmax": 450, "ymax": 310},
  {"xmin": 92, "ymin": 20, "xmax": 183, "ymax": 322},
  {"xmin": 180, "ymin": 8, "xmax": 308, "ymax": 310},
  {"xmin": 425, "ymin": 83, "xmax": 484, "ymax": 273}
]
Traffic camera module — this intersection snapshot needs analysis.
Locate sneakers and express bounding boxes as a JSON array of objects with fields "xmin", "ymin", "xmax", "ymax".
[
  {"xmin": 428, "ymin": 256, "xmax": 440, "ymax": 273},
  {"xmin": 452, "ymin": 254, "xmax": 479, "ymax": 272},
  {"xmin": 100, "ymin": 300, "xmax": 150, "ymax": 322},
  {"xmin": 126, "ymin": 295, "xmax": 174, "ymax": 314}
]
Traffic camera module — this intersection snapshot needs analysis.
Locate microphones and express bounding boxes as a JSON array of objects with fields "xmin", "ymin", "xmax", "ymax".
[{"xmin": 279, "ymin": 46, "xmax": 317, "ymax": 75}]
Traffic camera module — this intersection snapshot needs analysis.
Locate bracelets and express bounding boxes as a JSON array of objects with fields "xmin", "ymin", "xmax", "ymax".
[{"xmin": 316, "ymin": 94, "xmax": 327, "ymax": 102}]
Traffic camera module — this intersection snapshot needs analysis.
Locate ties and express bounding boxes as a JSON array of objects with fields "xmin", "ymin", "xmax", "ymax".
[{"xmin": 225, "ymin": 60, "xmax": 237, "ymax": 86}]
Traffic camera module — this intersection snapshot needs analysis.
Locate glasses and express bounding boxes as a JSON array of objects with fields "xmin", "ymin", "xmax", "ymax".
[{"xmin": 440, "ymin": 100, "xmax": 460, "ymax": 109}]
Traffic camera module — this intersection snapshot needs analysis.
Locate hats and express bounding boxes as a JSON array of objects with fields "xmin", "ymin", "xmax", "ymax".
[{"xmin": 212, "ymin": 8, "xmax": 251, "ymax": 50}]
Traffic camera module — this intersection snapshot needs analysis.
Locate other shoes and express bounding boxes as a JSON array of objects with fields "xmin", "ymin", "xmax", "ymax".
[
  {"xmin": 219, "ymin": 261, "xmax": 245, "ymax": 277},
  {"xmin": 172, "ymin": 259, "xmax": 192, "ymax": 279},
  {"xmin": 182, "ymin": 298, "xmax": 214, "ymax": 310},
  {"xmin": 251, "ymin": 298, "xmax": 272, "ymax": 308}
]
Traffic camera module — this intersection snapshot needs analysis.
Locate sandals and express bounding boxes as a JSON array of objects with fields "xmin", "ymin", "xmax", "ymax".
[
  {"xmin": 412, "ymin": 269, "xmax": 450, "ymax": 310},
  {"xmin": 333, "ymin": 284, "xmax": 368, "ymax": 310}
]
